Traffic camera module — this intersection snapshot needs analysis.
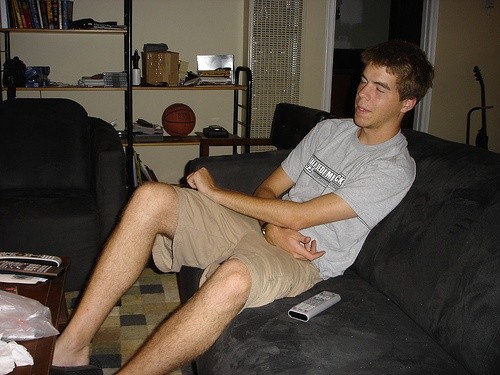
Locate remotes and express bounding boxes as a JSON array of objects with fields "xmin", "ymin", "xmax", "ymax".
[
  {"xmin": 0, "ymin": 252, "xmax": 66, "ymax": 278},
  {"xmin": 288, "ymin": 291, "xmax": 341, "ymax": 322}
]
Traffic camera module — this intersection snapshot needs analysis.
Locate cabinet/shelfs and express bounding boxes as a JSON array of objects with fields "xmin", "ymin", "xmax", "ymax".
[{"xmin": 0, "ymin": 0, "xmax": 252, "ymax": 184}]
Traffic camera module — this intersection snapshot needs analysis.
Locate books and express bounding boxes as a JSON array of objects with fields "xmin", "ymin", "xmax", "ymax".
[
  {"xmin": 132, "ymin": 118, "xmax": 164, "ymax": 138},
  {"xmin": 0, "ymin": 0, "xmax": 126, "ymax": 88},
  {"xmin": 197, "ymin": 70, "xmax": 231, "ymax": 84}
]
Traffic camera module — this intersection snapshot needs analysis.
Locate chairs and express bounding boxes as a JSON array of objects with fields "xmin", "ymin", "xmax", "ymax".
[{"xmin": 200, "ymin": 101, "xmax": 331, "ymax": 158}]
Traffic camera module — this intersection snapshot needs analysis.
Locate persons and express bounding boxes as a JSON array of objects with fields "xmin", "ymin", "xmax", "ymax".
[{"xmin": 52, "ymin": 40, "xmax": 435, "ymax": 375}]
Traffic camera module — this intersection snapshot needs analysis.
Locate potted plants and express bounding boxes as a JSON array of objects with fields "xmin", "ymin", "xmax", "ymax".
[{"xmin": 131, "ymin": 49, "xmax": 141, "ymax": 86}]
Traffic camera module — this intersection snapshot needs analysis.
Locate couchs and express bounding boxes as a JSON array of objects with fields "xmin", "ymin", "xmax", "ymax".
[
  {"xmin": 0, "ymin": 99, "xmax": 130, "ymax": 291},
  {"xmin": 177, "ymin": 128, "xmax": 500, "ymax": 375}
]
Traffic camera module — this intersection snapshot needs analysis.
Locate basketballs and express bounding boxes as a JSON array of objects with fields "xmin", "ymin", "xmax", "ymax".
[{"xmin": 162, "ymin": 104, "xmax": 195, "ymax": 136}]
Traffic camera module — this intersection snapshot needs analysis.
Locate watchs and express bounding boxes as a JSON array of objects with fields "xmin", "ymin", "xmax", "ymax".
[{"xmin": 261, "ymin": 222, "xmax": 269, "ymax": 235}]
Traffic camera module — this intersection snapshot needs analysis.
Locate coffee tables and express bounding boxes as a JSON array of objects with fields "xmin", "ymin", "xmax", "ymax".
[{"xmin": 0, "ymin": 256, "xmax": 70, "ymax": 375}]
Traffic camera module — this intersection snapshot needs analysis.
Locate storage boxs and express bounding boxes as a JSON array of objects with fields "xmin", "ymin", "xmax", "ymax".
[{"xmin": 140, "ymin": 49, "xmax": 180, "ymax": 86}]
